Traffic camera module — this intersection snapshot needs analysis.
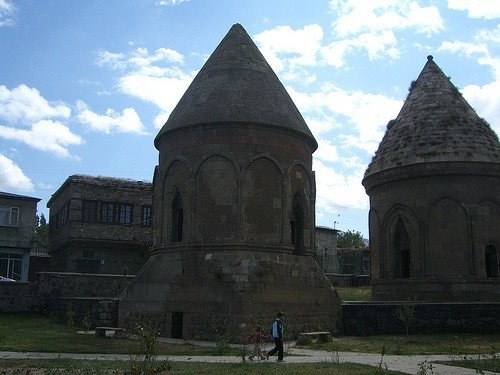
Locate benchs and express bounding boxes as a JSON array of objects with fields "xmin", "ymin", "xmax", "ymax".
[
  {"xmin": 95, "ymin": 327, "xmax": 126, "ymax": 338},
  {"xmin": 300, "ymin": 332, "xmax": 330, "ymax": 342}
]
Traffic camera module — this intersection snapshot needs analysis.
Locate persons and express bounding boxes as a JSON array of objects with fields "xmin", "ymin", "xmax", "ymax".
[
  {"xmin": 247, "ymin": 324, "xmax": 266, "ymax": 362},
  {"xmin": 265, "ymin": 310, "xmax": 286, "ymax": 363}
]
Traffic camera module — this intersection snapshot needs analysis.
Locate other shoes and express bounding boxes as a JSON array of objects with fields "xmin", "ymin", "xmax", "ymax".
[
  {"xmin": 249, "ymin": 355, "xmax": 254, "ymax": 361},
  {"xmin": 264, "ymin": 352, "xmax": 270, "ymax": 360},
  {"xmin": 276, "ymin": 359, "xmax": 286, "ymax": 362},
  {"xmin": 261, "ymin": 357, "xmax": 266, "ymax": 360}
]
